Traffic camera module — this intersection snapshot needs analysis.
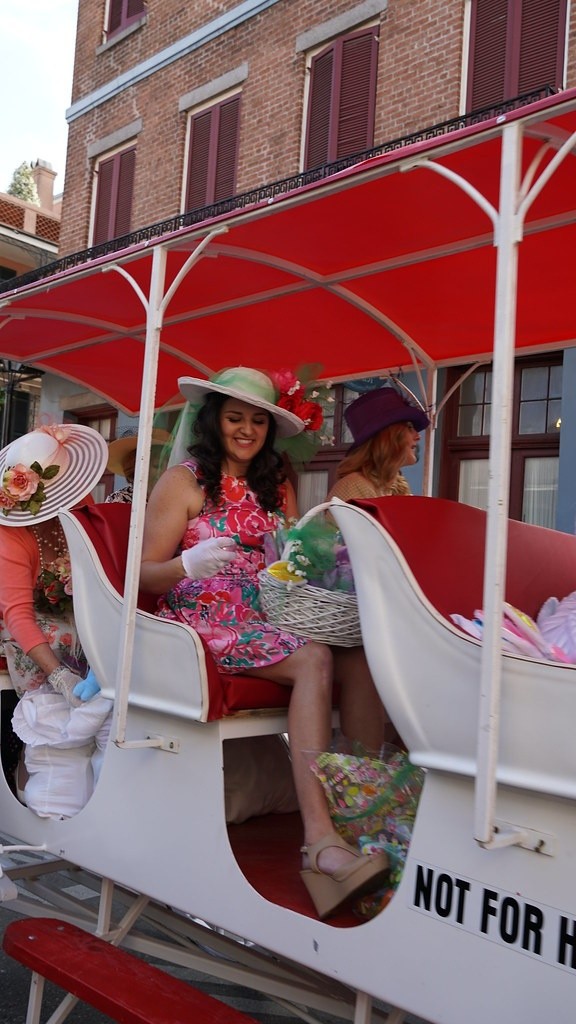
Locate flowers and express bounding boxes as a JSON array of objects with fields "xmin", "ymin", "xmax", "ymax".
[
  {"xmin": 273, "ymin": 366, "xmax": 336, "ymax": 447},
  {"xmin": 267, "ymin": 508, "xmax": 356, "ymax": 593},
  {"xmin": 39, "ymin": 421, "xmax": 73, "ymax": 442},
  {"xmin": 0, "ymin": 460, "xmax": 61, "ymax": 517}
]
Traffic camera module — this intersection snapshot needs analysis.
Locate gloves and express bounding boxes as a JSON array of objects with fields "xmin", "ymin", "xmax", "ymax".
[
  {"xmin": 181, "ymin": 537, "xmax": 235, "ymax": 581},
  {"xmin": 48, "ymin": 665, "xmax": 85, "ymax": 708}
]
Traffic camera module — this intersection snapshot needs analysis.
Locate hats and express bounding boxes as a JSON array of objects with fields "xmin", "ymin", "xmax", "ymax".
[
  {"xmin": 106, "ymin": 428, "xmax": 174, "ymax": 476},
  {"xmin": 0, "ymin": 422, "xmax": 108, "ymax": 526},
  {"xmin": 342, "ymin": 387, "xmax": 431, "ymax": 457},
  {"xmin": 177, "ymin": 367, "xmax": 323, "ymax": 439}
]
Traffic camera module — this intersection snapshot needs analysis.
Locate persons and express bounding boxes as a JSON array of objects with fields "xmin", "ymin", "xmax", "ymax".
[
  {"xmin": 0, "ymin": 424, "xmax": 110, "ymax": 806},
  {"xmin": 103, "ymin": 428, "xmax": 174, "ymax": 504},
  {"xmin": 138, "ymin": 367, "xmax": 393, "ymax": 916},
  {"xmin": 322, "ymin": 384, "xmax": 430, "ymax": 525}
]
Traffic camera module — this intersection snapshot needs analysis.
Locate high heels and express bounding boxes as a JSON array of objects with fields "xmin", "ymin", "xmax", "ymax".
[{"xmin": 299, "ymin": 833, "xmax": 391, "ymax": 918}]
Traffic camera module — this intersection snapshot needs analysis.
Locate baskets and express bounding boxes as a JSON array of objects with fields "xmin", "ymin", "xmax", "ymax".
[{"xmin": 256, "ymin": 502, "xmax": 365, "ymax": 648}]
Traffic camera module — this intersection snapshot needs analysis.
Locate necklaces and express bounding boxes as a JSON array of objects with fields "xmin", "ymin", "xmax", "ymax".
[{"xmin": 32, "ymin": 516, "xmax": 67, "ymax": 578}]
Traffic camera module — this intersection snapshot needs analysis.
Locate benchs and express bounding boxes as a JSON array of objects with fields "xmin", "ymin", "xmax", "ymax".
[
  {"xmin": 56, "ymin": 501, "xmax": 396, "ymax": 837},
  {"xmin": 327, "ymin": 495, "xmax": 576, "ymax": 921}
]
